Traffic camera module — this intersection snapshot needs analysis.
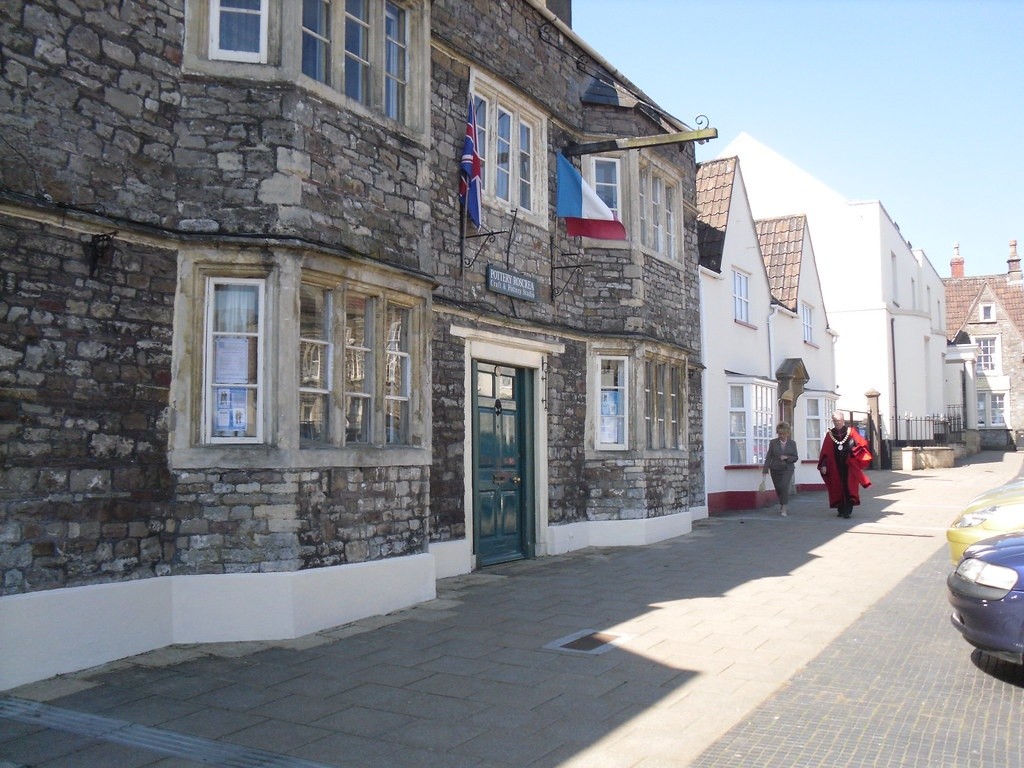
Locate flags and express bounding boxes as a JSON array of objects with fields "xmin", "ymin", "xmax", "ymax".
[
  {"xmin": 557, "ymin": 152, "xmax": 629, "ymax": 243},
  {"xmin": 459, "ymin": 102, "xmax": 483, "ymax": 230}
]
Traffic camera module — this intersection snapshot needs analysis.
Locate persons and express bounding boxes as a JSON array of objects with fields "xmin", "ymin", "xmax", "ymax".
[
  {"xmin": 817, "ymin": 410, "xmax": 874, "ymax": 519},
  {"xmin": 762, "ymin": 421, "xmax": 799, "ymax": 517}
]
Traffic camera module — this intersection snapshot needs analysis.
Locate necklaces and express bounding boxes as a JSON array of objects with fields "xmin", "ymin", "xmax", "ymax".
[
  {"xmin": 780, "ymin": 440, "xmax": 786, "ymax": 444},
  {"xmin": 829, "ymin": 427, "xmax": 851, "ymax": 450}
]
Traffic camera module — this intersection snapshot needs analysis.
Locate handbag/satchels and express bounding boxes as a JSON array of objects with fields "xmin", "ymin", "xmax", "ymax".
[{"xmin": 758, "ymin": 477, "xmax": 766, "ymax": 495}]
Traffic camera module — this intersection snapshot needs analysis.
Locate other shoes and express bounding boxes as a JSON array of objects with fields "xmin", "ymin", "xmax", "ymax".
[
  {"xmin": 779, "ymin": 507, "xmax": 782, "ymax": 513},
  {"xmin": 836, "ymin": 512, "xmax": 851, "ymax": 519},
  {"xmin": 780, "ymin": 511, "xmax": 786, "ymax": 517}
]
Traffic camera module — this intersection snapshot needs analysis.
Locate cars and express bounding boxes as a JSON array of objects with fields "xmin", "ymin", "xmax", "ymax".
[
  {"xmin": 947, "ymin": 530, "xmax": 1023, "ymax": 666},
  {"xmin": 947, "ymin": 477, "xmax": 1024, "ymax": 568}
]
